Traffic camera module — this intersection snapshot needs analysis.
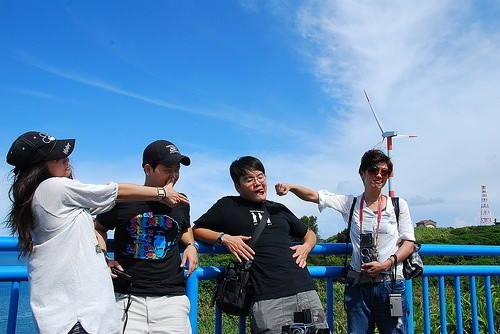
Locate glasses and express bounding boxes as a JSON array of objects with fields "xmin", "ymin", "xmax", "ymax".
[
  {"xmin": 364, "ymin": 166, "xmax": 391, "ymax": 178},
  {"xmin": 238, "ymin": 173, "xmax": 266, "ymax": 184}
]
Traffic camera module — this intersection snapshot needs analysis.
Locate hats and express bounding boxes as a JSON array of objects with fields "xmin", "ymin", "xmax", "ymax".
[
  {"xmin": 6, "ymin": 131, "xmax": 76, "ymax": 166},
  {"xmin": 142, "ymin": 140, "xmax": 191, "ymax": 168}
]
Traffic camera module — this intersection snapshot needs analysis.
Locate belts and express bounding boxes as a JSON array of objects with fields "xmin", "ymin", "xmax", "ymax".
[{"xmin": 346, "ymin": 269, "xmax": 402, "ymax": 283}]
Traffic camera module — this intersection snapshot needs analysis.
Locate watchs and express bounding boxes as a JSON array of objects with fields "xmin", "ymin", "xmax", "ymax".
[
  {"xmin": 158, "ymin": 187, "xmax": 166, "ymax": 201},
  {"xmin": 216, "ymin": 233, "xmax": 224, "ymax": 244}
]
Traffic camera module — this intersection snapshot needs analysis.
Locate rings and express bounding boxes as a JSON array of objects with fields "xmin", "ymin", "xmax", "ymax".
[{"xmin": 174, "ymin": 202, "xmax": 178, "ymax": 205}]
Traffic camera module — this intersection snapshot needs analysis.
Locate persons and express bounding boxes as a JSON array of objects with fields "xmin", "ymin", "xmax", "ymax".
[
  {"xmin": 275, "ymin": 149, "xmax": 416, "ymax": 334},
  {"xmin": 4, "ymin": 131, "xmax": 189, "ymax": 334},
  {"xmin": 192, "ymin": 156, "xmax": 330, "ymax": 334},
  {"xmin": 94, "ymin": 140, "xmax": 199, "ymax": 334}
]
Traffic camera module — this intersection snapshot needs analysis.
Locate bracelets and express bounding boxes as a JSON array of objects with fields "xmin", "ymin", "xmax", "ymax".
[
  {"xmin": 387, "ymin": 258, "xmax": 394, "ymax": 266},
  {"xmin": 189, "ymin": 243, "xmax": 198, "ymax": 250}
]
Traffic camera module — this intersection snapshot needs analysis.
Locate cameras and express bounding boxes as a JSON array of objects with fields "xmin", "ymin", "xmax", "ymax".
[
  {"xmin": 282, "ymin": 309, "xmax": 330, "ymax": 334},
  {"xmin": 360, "ymin": 232, "xmax": 375, "ymax": 265},
  {"xmin": 111, "ymin": 267, "xmax": 134, "ymax": 282}
]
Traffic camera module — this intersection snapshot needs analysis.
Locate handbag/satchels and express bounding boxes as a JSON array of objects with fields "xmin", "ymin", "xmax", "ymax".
[
  {"xmin": 216, "ymin": 261, "xmax": 252, "ymax": 317},
  {"xmin": 392, "ymin": 196, "xmax": 423, "ymax": 279}
]
